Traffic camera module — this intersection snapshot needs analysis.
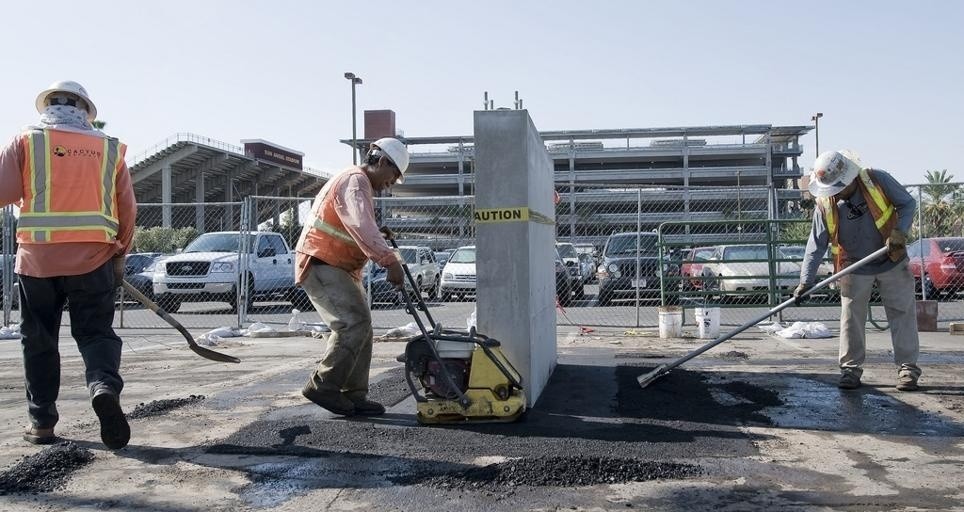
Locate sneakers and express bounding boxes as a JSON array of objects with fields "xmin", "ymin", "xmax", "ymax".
[
  {"xmin": 896, "ymin": 375, "xmax": 919, "ymax": 391},
  {"xmin": 837, "ymin": 375, "xmax": 862, "ymax": 389},
  {"xmin": 301, "ymin": 381, "xmax": 354, "ymax": 418},
  {"xmin": 344, "ymin": 391, "xmax": 386, "ymax": 416},
  {"xmin": 92, "ymin": 384, "xmax": 131, "ymax": 450},
  {"xmin": 23, "ymin": 423, "xmax": 55, "ymax": 444}
]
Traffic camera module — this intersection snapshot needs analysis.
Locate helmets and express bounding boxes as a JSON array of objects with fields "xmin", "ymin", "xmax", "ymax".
[
  {"xmin": 35, "ymin": 79, "xmax": 99, "ymax": 125},
  {"xmin": 368, "ymin": 136, "xmax": 410, "ymax": 185}
]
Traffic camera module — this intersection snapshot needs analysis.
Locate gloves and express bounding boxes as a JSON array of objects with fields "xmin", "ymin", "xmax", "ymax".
[
  {"xmin": 885, "ymin": 229, "xmax": 905, "ymax": 262},
  {"xmin": 794, "ymin": 284, "xmax": 811, "ymax": 306},
  {"xmin": 111, "ymin": 257, "xmax": 129, "ymax": 288}
]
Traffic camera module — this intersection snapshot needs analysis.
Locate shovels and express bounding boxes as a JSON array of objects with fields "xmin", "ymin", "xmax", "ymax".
[{"xmin": 14, "ymin": 201, "xmax": 241, "ymax": 363}]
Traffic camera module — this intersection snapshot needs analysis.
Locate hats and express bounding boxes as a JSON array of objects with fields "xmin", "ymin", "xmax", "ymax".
[{"xmin": 807, "ymin": 148, "xmax": 861, "ymax": 198}]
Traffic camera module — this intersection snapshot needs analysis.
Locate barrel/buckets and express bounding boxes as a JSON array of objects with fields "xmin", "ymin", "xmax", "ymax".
[
  {"xmin": 694, "ymin": 307, "xmax": 722, "ymax": 340},
  {"xmin": 658, "ymin": 311, "xmax": 682, "ymax": 340}
]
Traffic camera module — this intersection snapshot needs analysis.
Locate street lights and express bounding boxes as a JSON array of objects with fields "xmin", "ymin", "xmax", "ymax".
[
  {"xmin": 811, "ymin": 112, "xmax": 823, "ymax": 159},
  {"xmin": 344, "ymin": 71, "xmax": 362, "ymax": 166}
]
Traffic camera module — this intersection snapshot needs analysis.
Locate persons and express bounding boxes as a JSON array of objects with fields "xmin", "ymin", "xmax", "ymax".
[
  {"xmin": 791, "ymin": 149, "xmax": 922, "ymax": 392},
  {"xmin": 294, "ymin": 137, "xmax": 409, "ymax": 417},
  {"xmin": 0, "ymin": 81, "xmax": 137, "ymax": 450}
]
